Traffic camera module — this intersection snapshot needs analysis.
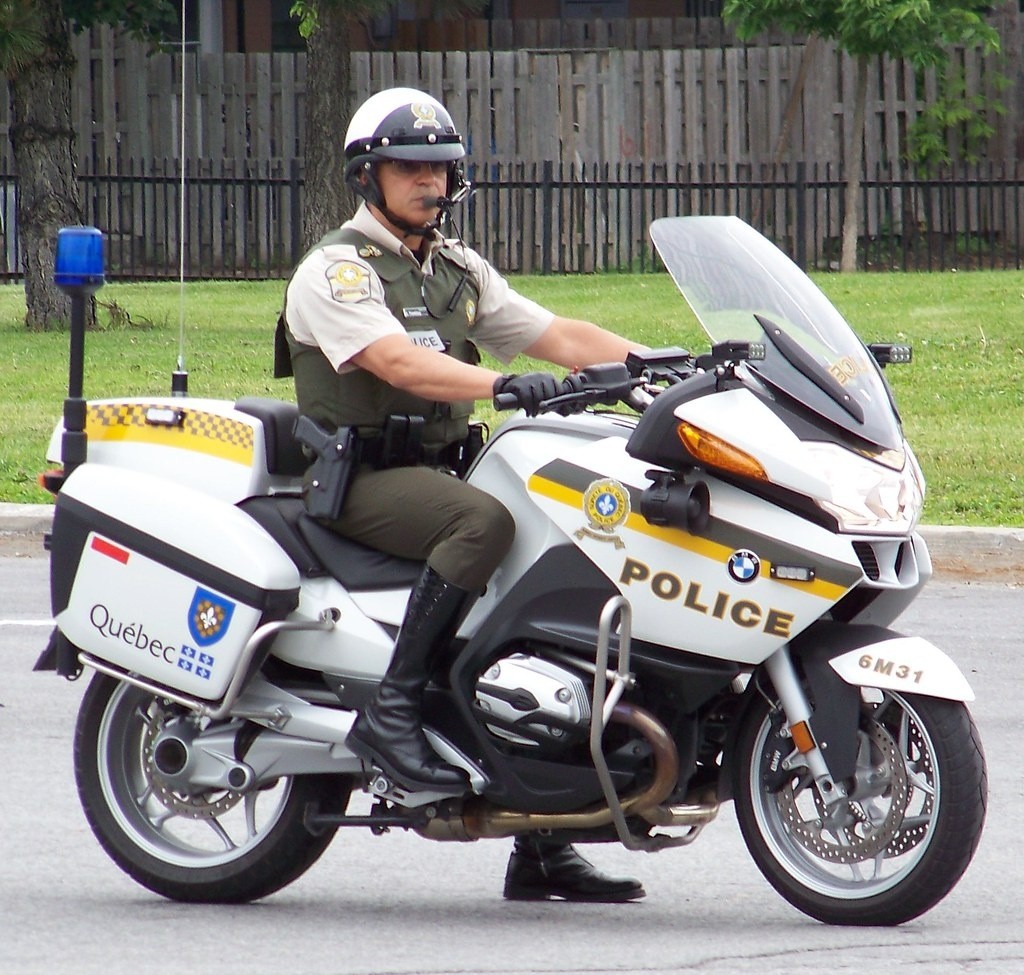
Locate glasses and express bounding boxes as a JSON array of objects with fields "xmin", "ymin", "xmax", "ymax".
[{"xmin": 375, "ymin": 155, "xmax": 456, "ymax": 177}]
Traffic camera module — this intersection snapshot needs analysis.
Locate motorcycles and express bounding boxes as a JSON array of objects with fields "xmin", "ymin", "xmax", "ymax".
[{"xmin": 30, "ymin": 214, "xmax": 988, "ymax": 927}]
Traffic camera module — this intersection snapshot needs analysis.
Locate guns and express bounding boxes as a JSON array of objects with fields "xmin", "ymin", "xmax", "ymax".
[{"xmin": 289, "ymin": 413, "xmax": 361, "ymax": 525}]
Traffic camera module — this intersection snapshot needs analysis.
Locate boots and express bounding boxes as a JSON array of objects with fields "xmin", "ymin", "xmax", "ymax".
[
  {"xmin": 503, "ymin": 833, "xmax": 646, "ymax": 901},
  {"xmin": 344, "ymin": 561, "xmax": 480, "ymax": 793}
]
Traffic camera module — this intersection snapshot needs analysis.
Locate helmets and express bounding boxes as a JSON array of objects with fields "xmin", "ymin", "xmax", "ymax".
[{"xmin": 343, "ymin": 87, "xmax": 465, "ymax": 183}]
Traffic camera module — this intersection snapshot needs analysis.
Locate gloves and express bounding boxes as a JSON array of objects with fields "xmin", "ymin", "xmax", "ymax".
[{"xmin": 493, "ymin": 372, "xmax": 564, "ymax": 418}]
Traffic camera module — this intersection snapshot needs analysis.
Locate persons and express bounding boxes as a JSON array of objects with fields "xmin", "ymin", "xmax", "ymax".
[{"xmin": 272, "ymin": 88, "xmax": 657, "ymax": 905}]
